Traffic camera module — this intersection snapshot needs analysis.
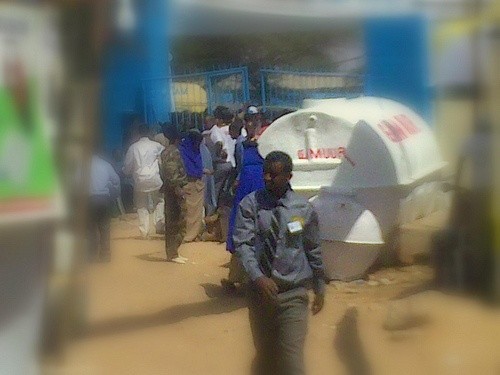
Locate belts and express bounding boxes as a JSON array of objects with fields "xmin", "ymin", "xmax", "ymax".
[{"xmin": 277, "ymin": 281, "xmax": 305, "ymax": 294}]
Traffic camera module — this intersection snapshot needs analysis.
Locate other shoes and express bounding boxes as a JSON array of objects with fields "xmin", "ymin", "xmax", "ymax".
[
  {"xmin": 220, "ymin": 277, "xmax": 258, "ymax": 294},
  {"xmin": 168, "ymin": 254, "xmax": 189, "ymax": 264}
]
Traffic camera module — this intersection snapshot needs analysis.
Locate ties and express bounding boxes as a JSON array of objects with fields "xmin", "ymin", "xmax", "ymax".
[{"xmin": 261, "ymin": 204, "xmax": 281, "ymax": 279}]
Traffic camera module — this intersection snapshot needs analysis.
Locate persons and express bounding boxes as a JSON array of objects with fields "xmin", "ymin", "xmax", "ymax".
[
  {"xmin": 160, "ymin": 130, "xmax": 189, "ymax": 264},
  {"xmin": 89, "ymin": 152, "xmax": 122, "ymax": 262},
  {"xmin": 122, "ymin": 125, "xmax": 166, "ymax": 242},
  {"xmin": 182, "ymin": 102, "xmax": 270, "ymax": 242},
  {"xmin": 233, "ymin": 151, "xmax": 327, "ymax": 375}
]
